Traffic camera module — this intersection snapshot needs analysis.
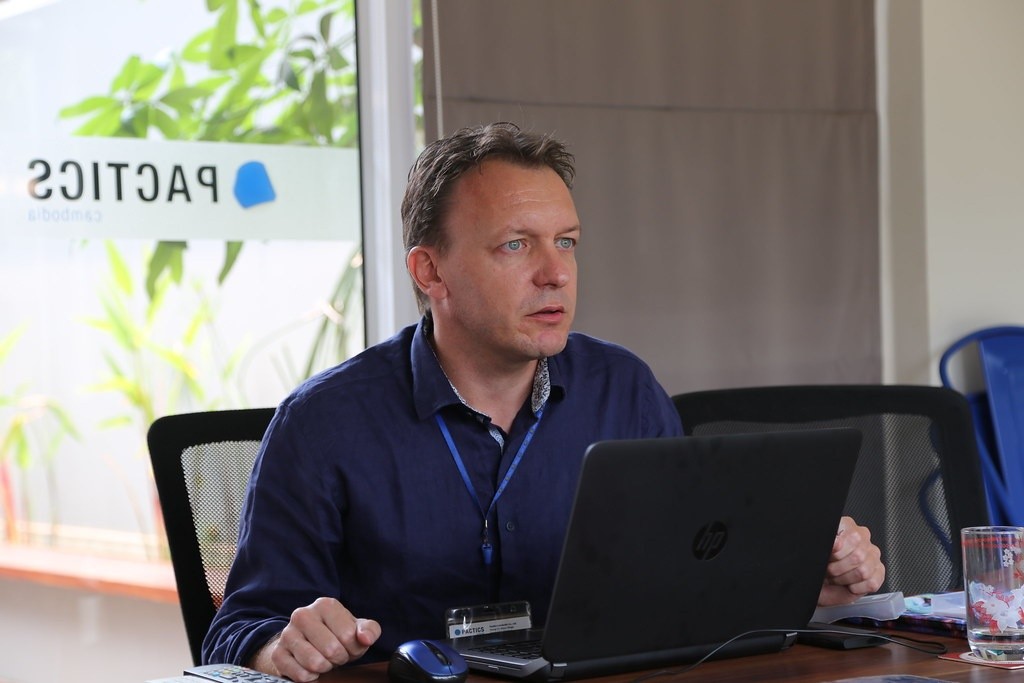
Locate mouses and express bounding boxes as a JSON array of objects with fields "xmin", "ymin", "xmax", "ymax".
[{"xmin": 394, "ymin": 638, "xmax": 469, "ymax": 683}]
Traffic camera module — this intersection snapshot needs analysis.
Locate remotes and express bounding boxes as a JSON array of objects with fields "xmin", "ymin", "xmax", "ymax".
[{"xmin": 148, "ymin": 663, "xmax": 294, "ymax": 683}]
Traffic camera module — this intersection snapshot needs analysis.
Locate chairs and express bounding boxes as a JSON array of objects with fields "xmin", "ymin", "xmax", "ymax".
[
  {"xmin": 147, "ymin": 406, "xmax": 276, "ymax": 666},
  {"xmin": 918, "ymin": 326, "xmax": 1024, "ymax": 561},
  {"xmin": 668, "ymin": 321, "xmax": 1024, "ymax": 597}
]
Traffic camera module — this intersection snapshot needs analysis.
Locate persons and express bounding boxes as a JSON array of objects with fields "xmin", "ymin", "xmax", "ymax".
[{"xmin": 201, "ymin": 121, "xmax": 886, "ymax": 683}]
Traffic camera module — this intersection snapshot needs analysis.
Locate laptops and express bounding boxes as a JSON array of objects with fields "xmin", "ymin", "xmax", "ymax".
[{"xmin": 453, "ymin": 426, "xmax": 865, "ymax": 683}]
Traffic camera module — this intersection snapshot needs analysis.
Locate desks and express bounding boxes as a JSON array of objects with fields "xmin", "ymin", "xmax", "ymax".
[{"xmin": 147, "ymin": 628, "xmax": 1024, "ymax": 683}]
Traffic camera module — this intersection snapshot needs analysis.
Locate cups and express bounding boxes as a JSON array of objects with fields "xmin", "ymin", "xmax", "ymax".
[{"xmin": 961, "ymin": 526, "xmax": 1024, "ymax": 661}]
